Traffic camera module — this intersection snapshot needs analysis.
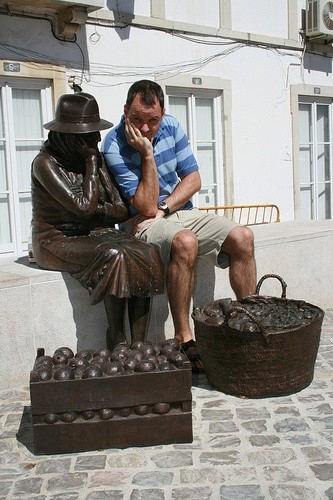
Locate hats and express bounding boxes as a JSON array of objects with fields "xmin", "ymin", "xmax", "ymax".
[{"xmin": 42, "ymin": 93, "xmax": 114, "ymax": 134}]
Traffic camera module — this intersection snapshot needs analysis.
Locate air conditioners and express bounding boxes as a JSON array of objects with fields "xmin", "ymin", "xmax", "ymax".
[{"xmin": 298, "ymin": 0, "xmax": 333, "ymax": 45}]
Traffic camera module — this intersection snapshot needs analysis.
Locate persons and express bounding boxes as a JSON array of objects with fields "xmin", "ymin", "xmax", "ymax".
[
  {"xmin": 32, "ymin": 93, "xmax": 165, "ymax": 351},
  {"xmin": 100, "ymin": 79, "xmax": 258, "ymax": 375}
]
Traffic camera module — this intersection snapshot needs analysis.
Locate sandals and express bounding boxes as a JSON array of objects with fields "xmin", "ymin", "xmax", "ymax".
[{"xmin": 180, "ymin": 339, "xmax": 206, "ymax": 373}]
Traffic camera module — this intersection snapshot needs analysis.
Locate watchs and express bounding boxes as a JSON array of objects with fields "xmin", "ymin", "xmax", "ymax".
[{"xmin": 158, "ymin": 201, "xmax": 170, "ymax": 215}]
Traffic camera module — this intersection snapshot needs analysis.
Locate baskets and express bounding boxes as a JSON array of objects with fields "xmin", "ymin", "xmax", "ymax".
[{"xmin": 192, "ymin": 273, "xmax": 325, "ymax": 399}]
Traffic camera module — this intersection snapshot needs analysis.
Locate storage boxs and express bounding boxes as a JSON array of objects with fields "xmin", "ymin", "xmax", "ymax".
[
  {"xmin": 29, "ymin": 348, "xmax": 192, "ymax": 452},
  {"xmin": 193, "ymin": 273, "xmax": 325, "ymax": 398}
]
